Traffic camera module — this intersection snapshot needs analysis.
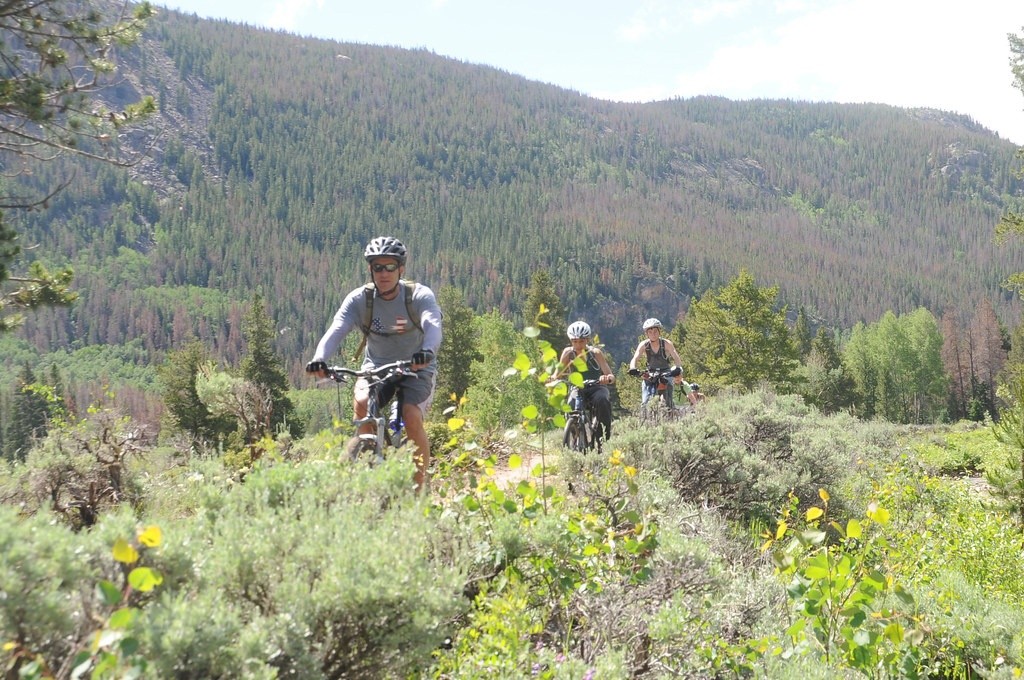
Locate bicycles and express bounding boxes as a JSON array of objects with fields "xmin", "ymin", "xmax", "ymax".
[
  {"xmin": 553, "ymin": 378, "xmax": 611, "ymax": 474},
  {"xmin": 633, "ymin": 369, "xmax": 679, "ymax": 427},
  {"xmin": 321, "ymin": 359, "xmax": 424, "ymax": 466}
]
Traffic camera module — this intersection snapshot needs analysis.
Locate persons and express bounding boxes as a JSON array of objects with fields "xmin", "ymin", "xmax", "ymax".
[
  {"xmin": 688, "ymin": 382, "xmax": 705, "ymax": 408},
  {"xmin": 630, "ymin": 317, "xmax": 684, "ymax": 424},
  {"xmin": 671, "ymin": 366, "xmax": 696, "ymax": 413},
  {"xmin": 545, "ymin": 320, "xmax": 616, "ymax": 440},
  {"xmin": 305, "ymin": 236, "xmax": 442, "ymax": 499}
]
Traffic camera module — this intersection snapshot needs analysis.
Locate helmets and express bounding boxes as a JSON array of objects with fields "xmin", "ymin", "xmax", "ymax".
[
  {"xmin": 642, "ymin": 318, "xmax": 662, "ymax": 331},
  {"xmin": 365, "ymin": 236, "xmax": 407, "ymax": 265},
  {"xmin": 690, "ymin": 383, "xmax": 699, "ymax": 390},
  {"xmin": 567, "ymin": 320, "xmax": 591, "ymax": 340},
  {"xmin": 671, "ymin": 366, "xmax": 684, "ymax": 374}
]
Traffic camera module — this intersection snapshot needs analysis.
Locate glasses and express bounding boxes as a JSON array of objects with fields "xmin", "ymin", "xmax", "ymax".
[{"xmin": 370, "ymin": 263, "xmax": 400, "ymax": 272}]
